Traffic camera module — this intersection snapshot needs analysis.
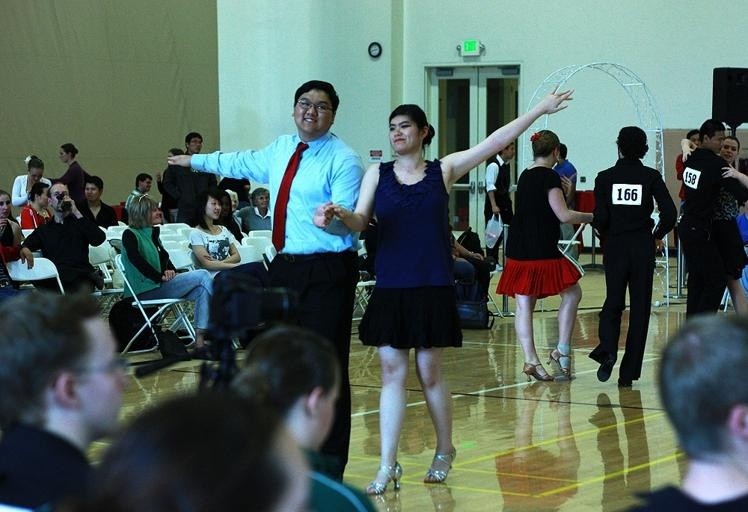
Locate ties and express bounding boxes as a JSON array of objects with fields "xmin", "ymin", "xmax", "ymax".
[{"xmin": 271, "ymin": 142, "xmax": 309, "ymax": 253}]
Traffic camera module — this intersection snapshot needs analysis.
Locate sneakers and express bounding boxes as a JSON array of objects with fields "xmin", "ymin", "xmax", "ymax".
[{"xmin": 496, "ymin": 264, "xmax": 503, "ymax": 270}]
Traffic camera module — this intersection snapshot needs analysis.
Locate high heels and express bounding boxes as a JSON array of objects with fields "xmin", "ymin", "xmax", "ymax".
[
  {"xmin": 364, "ymin": 461, "xmax": 402, "ymax": 493},
  {"xmin": 523, "ymin": 362, "xmax": 554, "ymax": 382},
  {"xmin": 423, "ymin": 482, "xmax": 456, "ymax": 512},
  {"xmin": 425, "ymin": 446, "xmax": 457, "ymax": 482},
  {"xmin": 523, "ymin": 380, "xmax": 555, "ymax": 399},
  {"xmin": 369, "ymin": 492, "xmax": 401, "ymax": 512},
  {"xmin": 546, "ymin": 376, "xmax": 575, "ymax": 409},
  {"xmin": 546, "ymin": 344, "xmax": 571, "ymax": 380}
]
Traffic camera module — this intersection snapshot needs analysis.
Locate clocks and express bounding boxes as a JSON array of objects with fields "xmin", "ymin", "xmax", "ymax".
[{"xmin": 368, "ymin": 43, "xmax": 382, "ymax": 58}]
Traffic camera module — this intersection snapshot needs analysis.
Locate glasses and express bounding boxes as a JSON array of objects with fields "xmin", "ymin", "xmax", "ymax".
[{"xmin": 297, "ymin": 98, "xmax": 334, "ymax": 113}]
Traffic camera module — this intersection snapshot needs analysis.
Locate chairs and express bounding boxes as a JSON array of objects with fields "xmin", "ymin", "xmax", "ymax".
[
  {"xmin": 165, "ymin": 249, "xmax": 196, "ymax": 339},
  {"xmin": 353, "ymin": 282, "xmax": 369, "ymax": 313},
  {"xmin": 6, "ymin": 258, "xmax": 65, "ymax": 296},
  {"xmin": 88, "ymin": 243, "xmax": 124, "ymax": 314},
  {"xmin": 115, "ymin": 254, "xmax": 195, "ymax": 356},
  {"xmin": 357, "ymin": 240, "xmax": 367, "ymax": 259},
  {"xmin": 154, "ymin": 223, "xmax": 194, "ymax": 253},
  {"xmin": 557, "ymin": 222, "xmax": 586, "ymax": 276},
  {"xmin": 488, "ymin": 270, "xmax": 504, "ymax": 318},
  {"xmin": 189, "ymin": 251, "xmax": 239, "ymax": 351},
  {"xmin": 357, "ymin": 281, "xmax": 376, "ymax": 305},
  {"xmin": 98, "ymin": 221, "xmax": 130, "ymax": 239},
  {"xmin": 236, "ymin": 230, "xmax": 277, "ymax": 271}
]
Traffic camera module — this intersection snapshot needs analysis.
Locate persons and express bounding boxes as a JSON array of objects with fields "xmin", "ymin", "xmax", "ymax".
[
  {"xmin": 21, "ymin": 183, "xmax": 106, "ymax": 292},
  {"xmin": 20, "ymin": 181, "xmax": 51, "ymax": 229},
  {"xmin": 588, "ymin": 126, "xmax": 677, "ymax": 387},
  {"xmin": 1, "ymin": 291, "xmax": 126, "ymax": 511},
  {"xmin": 238, "ymin": 187, "xmax": 270, "ymax": 232},
  {"xmin": 168, "ymin": 80, "xmax": 365, "ymax": 484},
  {"xmin": 211, "ymin": 188, "xmax": 241, "ymax": 245},
  {"xmin": 158, "ymin": 149, "xmax": 185, "ymax": 222},
  {"xmin": 190, "ymin": 191, "xmax": 242, "ymax": 279},
  {"xmin": 121, "ymin": 194, "xmax": 215, "ymax": 358},
  {"xmin": 676, "ymin": 119, "xmax": 748, "ymax": 319},
  {"xmin": 0, "ymin": 191, "xmax": 21, "ymax": 291},
  {"xmin": 69, "ymin": 393, "xmax": 307, "ymax": 512},
  {"xmin": 612, "ymin": 313, "xmax": 748, "ymax": 512},
  {"xmin": 496, "ymin": 130, "xmax": 593, "ymax": 382},
  {"xmin": 451, "ymin": 230, "xmax": 484, "ymax": 302},
  {"xmin": 327, "ymin": 83, "xmax": 574, "ymax": 496},
  {"xmin": 13, "ymin": 156, "xmax": 52, "ymax": 207},
  {"xmin": 126, "ymin": 173, "xmax": 153, "ymax": 208},
  {"xmin": 486, "ymin": 141, "xmax": 516, "ymax": 263},
  {"xmin": 231, "ymin": 326, "xmax": 378, "ymax": 511},
  {"xmin": 554, "ymin": 143, "xmax": 577, "ymax": 207},
  {"xmin": 48, "ymin": 142, "xmax": 91, "ymax": 200},
  {"xmin": 79, "ymin": 175, "xmax": 117, "ymax": 227},
  {"xmin": 179, "ymin": 132, "xmax": 217, "ymax": 224},
  {"xmin": 226, "ymin": 190, "xmax": 240, "ymax": 213}
]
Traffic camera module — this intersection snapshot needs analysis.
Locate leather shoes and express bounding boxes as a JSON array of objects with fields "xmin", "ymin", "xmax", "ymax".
[
  {"xmin": 597, "ymin": 353, "xmax": 617, "ymax": 383},
  {"xmin": 618, "ymin": 379, "xmax": 632, "ymax": 386}
]
